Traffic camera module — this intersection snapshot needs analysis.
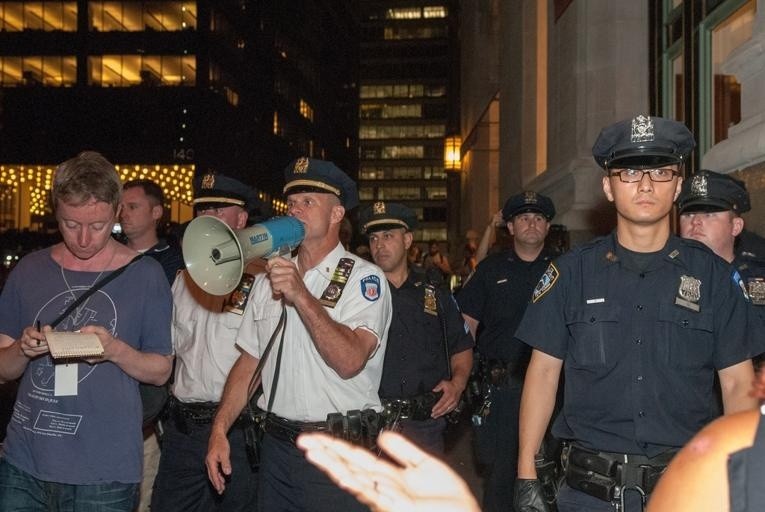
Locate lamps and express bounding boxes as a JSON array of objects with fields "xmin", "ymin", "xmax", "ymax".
[{"xmin": 441, "ymin": 133, "xmax": 462, "ymax": 176}]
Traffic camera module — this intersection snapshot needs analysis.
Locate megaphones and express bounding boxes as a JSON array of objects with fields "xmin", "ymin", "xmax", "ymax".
[{"xmin": 182, "ymin": 215, "xmax": 305, "ymax": 296}]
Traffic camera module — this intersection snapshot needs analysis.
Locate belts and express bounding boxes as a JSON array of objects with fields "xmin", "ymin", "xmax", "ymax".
[
  {"xmin": 164, "ymin": 392, "xmax": 246, "ymax": 427},
  {"xmin": 555, "ymin": 441, "xmax": 667, "ymax": 504},
  {"xmin": 476, "ymin": 358, "xmax": 538, "ymax": 386},
  {"xmin": 249, "ymin": 395, "xmax": 387, "ymax": 451},
  {"xmin": 383, "ymin": 388, "xmax": 453, "ymax": 416}
]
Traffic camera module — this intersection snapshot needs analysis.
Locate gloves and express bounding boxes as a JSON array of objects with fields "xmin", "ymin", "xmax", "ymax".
[{"xmin": 512, "ymin": 478, "xmax": 548, "ymax": 512}]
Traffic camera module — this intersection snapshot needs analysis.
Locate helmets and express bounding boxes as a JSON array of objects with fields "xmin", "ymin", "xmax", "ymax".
[{"xmin": 606, "ymin": 168, "xmax": 683, "ymax": 184}]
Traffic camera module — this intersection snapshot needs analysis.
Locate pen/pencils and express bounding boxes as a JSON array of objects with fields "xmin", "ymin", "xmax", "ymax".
[{"xmin": 37, "ymin": 320, "xmax": 41, "ymax": 346}]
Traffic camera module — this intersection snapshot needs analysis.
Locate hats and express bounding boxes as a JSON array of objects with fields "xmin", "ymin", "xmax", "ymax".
[
  {"xmin": 591, "ymin": 113, "xmax": 697, "ymax": 170},
  {"xmin": 360, "ymin": 201, "xmax": 419, "ymax": 235},
  {"xmin": 673, "ymin": 168, "xmax": 752, "ymax": 216},
  {"xmin": 191, "ymin": 173, "xmax": 257, "ymax": 212},
  {"xmin": 283, "ymin": 157, "xmax": 360, "ymax": 215},
  {"xmin": 502, "ymin": 190, "xmax": 557, "ymax": 224}
]
